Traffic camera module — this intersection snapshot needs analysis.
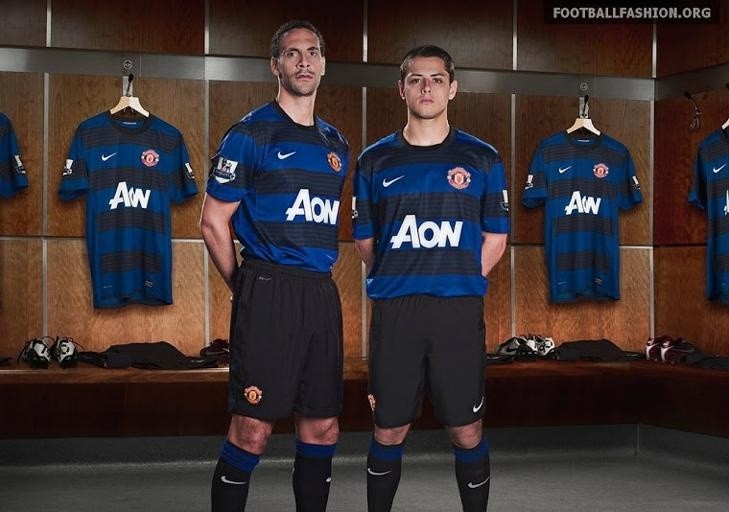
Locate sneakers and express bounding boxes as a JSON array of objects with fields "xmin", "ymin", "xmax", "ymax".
[
  {"xmin": 643, "ymin": 336, "xmax": 663, "ymax": 361},
  {"xmin": 526, "ymin": 333, "xmax": 559, "ymax": 361},
  {"xmin": 660, "ymin": 335, "xmax": 697, "ymax": 364},
  {"xmin": 486, "ymin": 334, "xmax": 546, "ymax": 364},
  {"xmin": 18, "ymin": 337, "xmax": 52, "ymax": 368},
  {"xmin": 50, "ymin": 335, "xmax": 80, "ymax": 369}
]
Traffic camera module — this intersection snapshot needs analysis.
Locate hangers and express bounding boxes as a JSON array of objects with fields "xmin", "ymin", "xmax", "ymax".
[
  {"xmin": 722, "ymin": 118, "xmax": 729, "ymax": 131},
  {"xmin": 566, "ymin": 93, "xmax": 601, "ymax": 136},
  {"xmin": 108, "ymin": 61, "xmax": 149, "ymax": 120}
]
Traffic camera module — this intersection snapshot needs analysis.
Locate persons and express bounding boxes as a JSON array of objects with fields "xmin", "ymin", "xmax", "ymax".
[
  {"xmin": 350, "ymin": 45, "xmax": 511, "ymax": 511},
  {"xmin": 198, "ymin": 21, "xmax": 351, "ymax": 512}
]
